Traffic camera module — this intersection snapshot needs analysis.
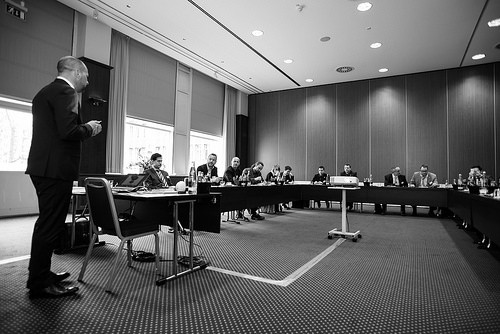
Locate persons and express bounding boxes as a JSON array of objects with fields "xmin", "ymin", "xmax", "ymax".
[
  {"xmin": 143, "ymin": 153, "xmax": 187, "ymax": 235},
  {"xmin": 454, "ymin": 167, "xmax": 499, "ymax": 248},
  {"xmin": 381, "ymin": 167, "xmax": 409, "ymax": 215},
  {"xmin": 339, "ymin": 164, "xmax": 361, "ymax": 211},
  {"xmin": 410, "ymin": 165, "xmax": 440, "ymax": 218},
  {"xmin": 310, "ymin": 164, "xmax": 332, "ymax": 209},
  {"xmin": 23, "ymin": 55, "xmax": 102, "ymax": 298},
  {"xmin": 196, "ymin": 152, "xmax": 295, "ymax": 222}
]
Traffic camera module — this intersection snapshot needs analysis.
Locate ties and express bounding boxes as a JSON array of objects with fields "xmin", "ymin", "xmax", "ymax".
[{"xmin": 156, "ymin": 170, "xmax": 169, "ymax": 188}]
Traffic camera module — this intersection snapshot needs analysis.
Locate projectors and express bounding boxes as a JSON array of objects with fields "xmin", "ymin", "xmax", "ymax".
[{"xmin": 330, "ymin": 176, "xmax": 359, "ymax": 186}]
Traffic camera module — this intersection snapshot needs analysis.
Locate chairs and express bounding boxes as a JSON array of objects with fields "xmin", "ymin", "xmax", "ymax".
[{"xmin": 77, "ymin": 177, "xmax": 161, "ymax": 292}]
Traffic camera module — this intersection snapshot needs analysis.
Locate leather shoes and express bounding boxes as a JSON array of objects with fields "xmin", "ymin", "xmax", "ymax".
[
  {"xmin": 28, "ymin": 283, "xmax": 79, "ymax": 300},
  {"xmin": 27, "ymin": 271, "xmax": 71, "ymax": 289}
]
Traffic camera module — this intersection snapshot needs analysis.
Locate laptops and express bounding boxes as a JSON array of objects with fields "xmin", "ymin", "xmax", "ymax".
[{"xmin": 112, "ymin": 174, "xmax": 150, "ymax": 191}]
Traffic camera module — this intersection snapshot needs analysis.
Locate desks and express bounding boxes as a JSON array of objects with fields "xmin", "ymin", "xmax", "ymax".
[
  {"xmin": 54, "ymin": 190, "xmax": 222, "ymax": 286},
  {"xmin": 210, "ymin": 181, "xmax": 500, "ymax": 248}
]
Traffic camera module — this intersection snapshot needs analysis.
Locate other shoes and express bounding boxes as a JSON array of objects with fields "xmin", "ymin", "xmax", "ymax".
[
  {"xmin": 349, "ymin": 205, "xmax": 353, "ymax": 210},
  {"xmin": 282, "ymin": 204, "xmax": 287, "ymax": 208},
  {"xmin": 237, "ymin": 215, "xmax": 249, "ymax": 222},
  {"xmin": 381, "ymin": 210, "xmax": 385, "ymax": 215},
  {"xmin": 374, "ymin": 211, "xmax": 381, "ymax": 214},
  {"xmin": 318, "ymin": 204, "xmax": 321, "ymax": 208},
  {"xmin": 251, "ymin": 214, "xmax": 265, "ymax": 220},
  {"xmin": 428, "ymin": 212, "xmax": 435, "ymax": 217},
  {"xmin": 412, "ymin": 212, "xmax": 417, "ymax": 216},
  {"xmin": 327, "ymin": 202, "xmax": 329, "ymax": 208}
]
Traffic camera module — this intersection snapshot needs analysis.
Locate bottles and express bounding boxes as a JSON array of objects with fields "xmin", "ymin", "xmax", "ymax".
[
  {"xmin": 188, "ymin": 160, "xmax": 204, "ymax": 187},
  {"xmin": 232, "ymin": 170, "xmax": 251, "ymax": 186},
  {"xmin": 322, "ymin": 173, "xmax": 329, "ymax": 185},
  {"xmin": 363, "ymin": 174, "xmax": 374, "ymax": 186}
]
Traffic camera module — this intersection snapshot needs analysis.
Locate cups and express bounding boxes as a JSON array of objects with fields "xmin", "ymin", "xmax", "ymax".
[
  {"xmin": 239, "ymin": 181, "xmax": 250, "ymax": 186},
  {"xmin": 220, "ymin": 181, "xmax": 225, "ymax": 187},
  {"xmin": 363, "ymin": 181, "xmax": 373, "ymax": 186}
]
